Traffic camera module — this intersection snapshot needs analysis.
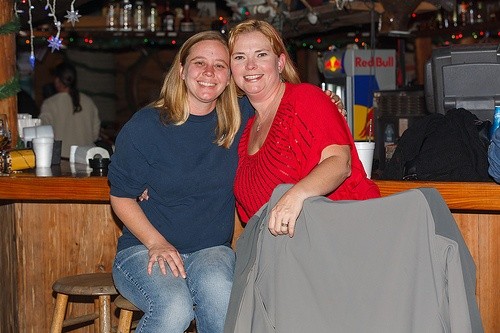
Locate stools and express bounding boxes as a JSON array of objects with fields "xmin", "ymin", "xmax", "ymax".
[
  {"xmin": 114, "ymin": 295, "xmax": 195, "ymax": 333},
  {"xmin": 51, "ymin": 273, "xmax": 119, "ymax": 333}
]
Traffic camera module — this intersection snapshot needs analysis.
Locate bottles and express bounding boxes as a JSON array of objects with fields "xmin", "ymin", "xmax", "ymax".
[
  {"xmin": 487, "ymin": 99, "xmax": 500, "ymax": 142},
  {"xmin": 146, "ymin": 0, "xmax": 161, "ymax": 32},
  {"xmin": 161, "ymin": 0, "xmax": 175, "ymax": 32},
  {"xmin": 196, "ymin": 4, "xmax": 212, "ymax": 32},
  {"xmin": 104, "ymin": 3, "xmax": 119, "ymax": 32},
  {"xmin": 133, "ymin": 0, "xmax": 146, "ymax": 32},
  {"xmin": 436, "ymin": 0, "xmax": 498, "ymax": 31},
  {"xmin": 179, "ymin": 4, "xmax": 195, "ymax": 33},
  {"xmin": 119, "ymin": 0, "xmax": 132, "ymax": 32},
  {"xmin": 0, "ymin": 113, "xmax": 13, "ymax": 177}
]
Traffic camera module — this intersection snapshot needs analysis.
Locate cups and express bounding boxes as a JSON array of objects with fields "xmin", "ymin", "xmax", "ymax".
[
  {"xmin": 354, "ymin": 141, "xmax": 375, "ymax": 180},
  {"xmin": 33, "ymin": 138, "xmax": 54, "ymax": 168},
  {"xmin": 52, "ymin": 139, "xmax": 63, "ymax": 166}
]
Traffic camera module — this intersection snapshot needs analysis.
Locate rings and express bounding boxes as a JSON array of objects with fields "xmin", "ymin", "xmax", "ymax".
[
  {"xmin": 282, "ymin": 223, "xmax": 288, "ymax": 227},
  {"xmin": 157, "ymin": 257, "xmax": 163, "ymax": 261}
]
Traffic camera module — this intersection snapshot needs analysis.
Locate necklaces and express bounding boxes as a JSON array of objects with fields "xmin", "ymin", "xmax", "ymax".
[{"xmin": 256, "ymin": 109, "xmax": 268, "ymax": 131}]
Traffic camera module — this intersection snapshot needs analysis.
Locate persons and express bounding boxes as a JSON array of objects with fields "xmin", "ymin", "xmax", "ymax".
[
  {"xmin": 109, "ymin": 32, "xmax": 348, "ymax": 333},
  {"xmin": 137, "ymin": 20, "xmax": 382, "ymax": 237},
  {"xmin": 37, "ymin": 60, "xmax": 101, "ymax": 160}
]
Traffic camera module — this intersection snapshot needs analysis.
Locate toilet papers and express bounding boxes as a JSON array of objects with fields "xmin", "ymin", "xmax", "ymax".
[
  {"xmin": 75, "ymin": 145, "xmax": 110, "ymax": 165},
  {"xmin": 69, "ymin": 145, "xmax": 78, "ymax": 163}
]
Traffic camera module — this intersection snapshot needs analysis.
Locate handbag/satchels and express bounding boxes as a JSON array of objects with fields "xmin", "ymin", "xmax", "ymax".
[{"xmin": 375, "ymin": 107, "xmax": 494, "ymax": 182}]
